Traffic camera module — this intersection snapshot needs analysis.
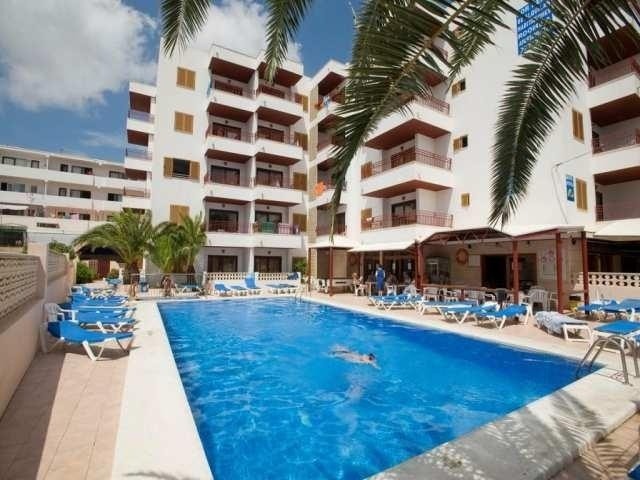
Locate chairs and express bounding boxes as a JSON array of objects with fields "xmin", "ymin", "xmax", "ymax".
[
  {"xmin": 38, "ymin": 281, "xmax": 137, "ymax": 362},
  {"xmin": 353, "ymin": 281, "xmax": 639, "ymax": 356},
  {"xmin": 141, "ymin": 275, "xmax": 300, "ymax": 296}
]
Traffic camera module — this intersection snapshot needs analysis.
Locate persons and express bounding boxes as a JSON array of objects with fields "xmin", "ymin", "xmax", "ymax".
[
  {"xmin": 329, "ymin": 344, "xmax": 383, "ymax": 369},
  {"xmin": 351, "ymin": 264, "xmax": 415, "ymax": 300},
  {"xmin": 160, "ymin": 274, "xmax": 173, "ymax": 299},
  {"xmin": 128, "ymin": 280, "xmax": 141, "ymax": 301},
  {"xmin": 195, "ymin": 276, "xmax": 212, "ymax": 300}
]
